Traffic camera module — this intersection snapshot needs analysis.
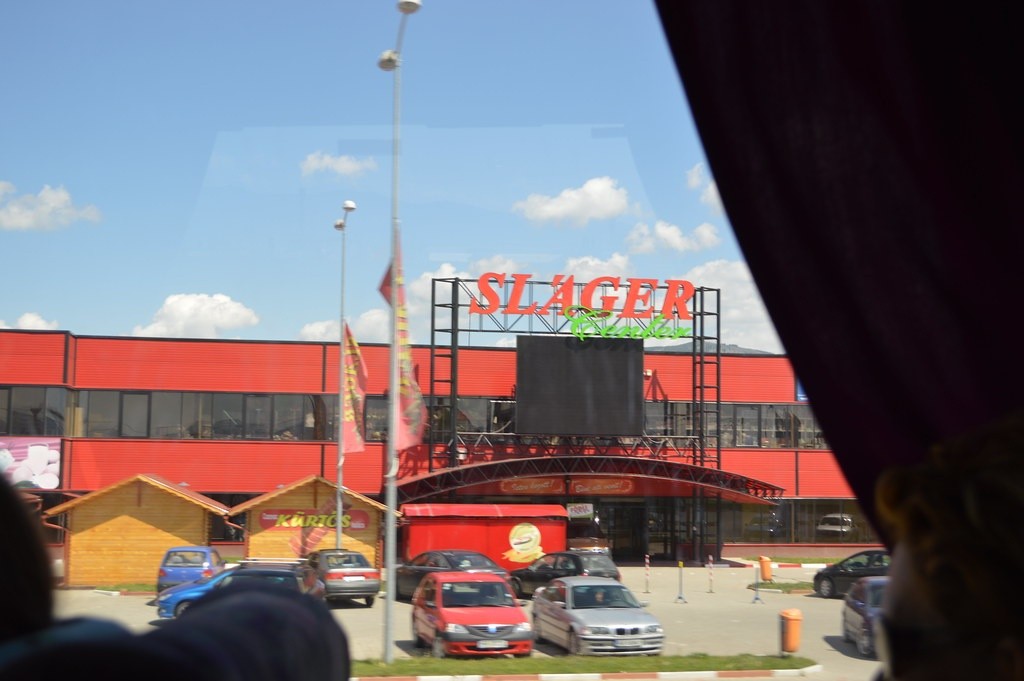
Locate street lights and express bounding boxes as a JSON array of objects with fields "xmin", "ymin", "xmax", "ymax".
[
  {"xmin": 334, "ymin": 200, "xmax": 358, "ymax": 560},
  {"xmin": 376, "ymin": 1, "xmax": 423, "ymax": 668}
]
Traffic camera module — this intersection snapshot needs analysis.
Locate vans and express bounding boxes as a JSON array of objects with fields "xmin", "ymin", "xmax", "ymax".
[{"xmin": 565, "ymin": 518, "xmax": 613, "ymax": 563}]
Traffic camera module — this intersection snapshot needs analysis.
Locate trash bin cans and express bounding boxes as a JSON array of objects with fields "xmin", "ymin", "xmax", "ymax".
[
  {"xmin": 759, "ymin": 556, "xmax": 773, "ymax": 582},
  {"xmin": 779, "ymin": 608, "xmax": 802, "ymax": 655}
]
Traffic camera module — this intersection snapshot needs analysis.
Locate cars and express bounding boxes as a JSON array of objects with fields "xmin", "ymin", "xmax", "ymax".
[
  {"xmin": 743, "ymin": 513, "xmax": 788, "ymax": 543},
  {"xmin": 396, "ymin": 548, "xmax": 513, "ymax": 606},
  {"xmin": 842, "ymin": 576, "xmax": 904, "ymax": 658},
  {"xmin": 410, "ymin": 570, "xmax": 534, "ymax": 659},
  {"xmin": 530, "ymin": 575, "xmax": 666, "ymax": 657},
  {"xmin": 510, "ymin": 549, "xmax": 622, "ymax": 603},
  {"xmin": 300, "ymin": 547, "xmax": 381, "ymax": 609},
  {"xmin": 155, "ymin": 546, "xmax": 228, "ymax": 595},
  {"xmin": 814, "ymin": 549, "xmax": 894, "ymax": 598}
]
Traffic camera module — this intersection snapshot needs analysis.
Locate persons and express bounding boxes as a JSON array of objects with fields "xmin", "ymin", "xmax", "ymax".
[
  {"xmin": 0, "ymin": 468, "xmax": 138, "ymax": 659},
  {"xmin": 879, "ymin": 407, "xmax": 1024, "ymax": 680}
]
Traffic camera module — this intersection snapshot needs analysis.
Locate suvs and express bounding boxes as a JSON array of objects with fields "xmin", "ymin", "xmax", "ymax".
[
  {"xmin": 158, "ymin": 563, "xmax": 329, "ymax": 623},
  {"xmin": 813, "ymin": 512, "xmax": 857, "ymax": 538}
]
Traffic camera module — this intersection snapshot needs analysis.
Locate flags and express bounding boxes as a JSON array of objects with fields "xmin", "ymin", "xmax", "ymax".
[
  {"xmin": 377, "ymin": 256, "xmax": 424, "ymax": 452},
  {"xmin": 339, "ymin": 321, "xmax": 371, "ymax": 453}
]
